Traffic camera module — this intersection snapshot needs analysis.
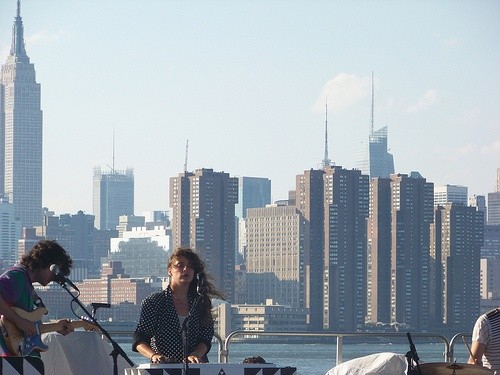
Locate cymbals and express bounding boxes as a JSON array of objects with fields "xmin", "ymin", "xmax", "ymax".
[{"xmin": 414, "ymin": 362, "xmax": 494, "ymax": 375}]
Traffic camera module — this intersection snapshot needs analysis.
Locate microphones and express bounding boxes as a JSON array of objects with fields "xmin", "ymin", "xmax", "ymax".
[
  {"xmin": 197, "ymin": 272, "xmax": 205, "ymax": 293},
  {"xmin": 50, "ymin": 264, "xmax": 80, "ymax": 292}
]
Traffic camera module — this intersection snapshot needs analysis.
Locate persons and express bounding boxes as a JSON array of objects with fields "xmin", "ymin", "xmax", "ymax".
[
  {"xmin": 467, "ymin": 307, "xmax": 500, "ymax": 375},
  {"xmin": 131, "ymin": 247, "xmax": 226, "ymax": 363},
  {"xmin": 0, "ymin": 239, "xmax": 74, "ymax": 356}
]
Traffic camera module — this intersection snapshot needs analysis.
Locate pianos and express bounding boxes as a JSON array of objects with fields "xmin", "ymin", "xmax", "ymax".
[{"xmin": 124, "ymin": 363, "xmax": 297, "ymax": 375}]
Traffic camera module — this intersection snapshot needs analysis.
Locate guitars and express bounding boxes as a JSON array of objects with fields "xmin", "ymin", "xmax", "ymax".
[{"xmin": 1, "ymin": 307, "xmax": 99, "ymax": 357}]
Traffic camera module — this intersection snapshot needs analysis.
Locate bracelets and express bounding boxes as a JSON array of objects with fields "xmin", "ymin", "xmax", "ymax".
[{"xmin": 150, "ymin": 352, "xmax": 158, "ymax": 362}]
